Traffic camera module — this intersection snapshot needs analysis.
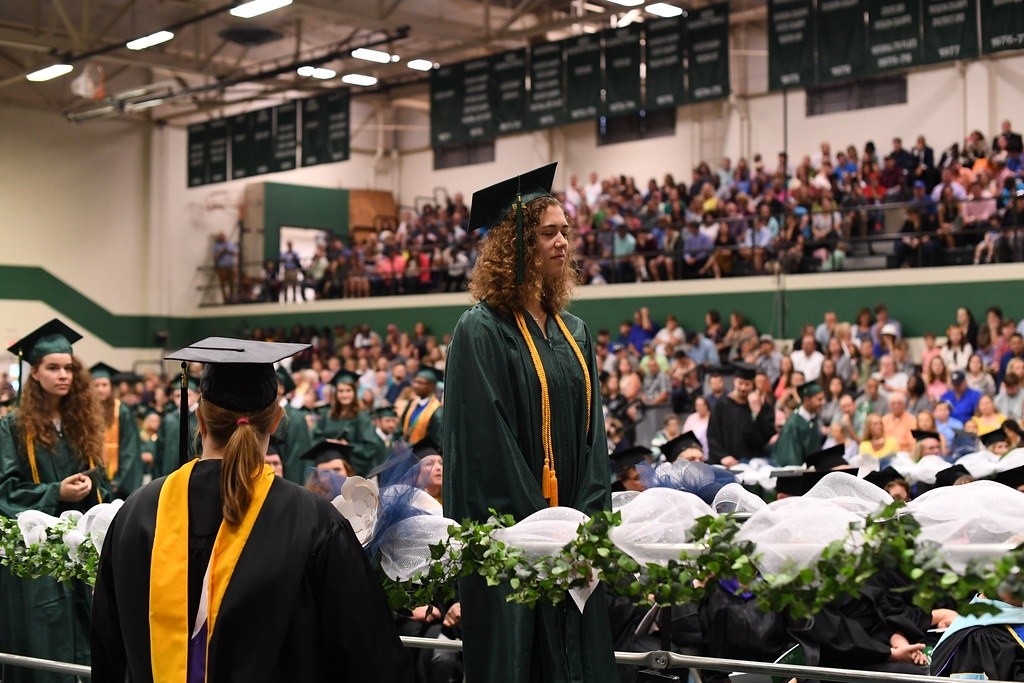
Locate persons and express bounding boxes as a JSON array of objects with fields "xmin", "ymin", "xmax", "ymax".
[
  {"xmin": 444, "ymin": 162, "xmax": 620, "ymax": 683},
  {"xmin": 92, "ymin": 336, "xmax": 415, "ymax": 683},
  {"xmin": 1, "ymin": 320, "xmax": 112, "ymax": 683},
  {"xmin": 0, "ymin": 120, "xmax": 1024, "ymax": 683}
]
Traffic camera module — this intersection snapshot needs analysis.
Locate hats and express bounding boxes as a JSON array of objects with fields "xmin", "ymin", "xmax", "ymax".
[
  {"xmin": 760, "ymin": 334, "xmax": 773, "ymax": 343},
  {"xmin": 981, "ymin": 428, "xmax": 1005, "ymax": 447},
  {"xmin": 7, "ymin": 318, "xmax": 83, "ymax": 406},
  {"xmin": 770, "ymin": 468, "xmax": 860, "ymax": 494},
  {"xmin": 731, "ymin": 362, "xmax": 756, "ymax": 380},
  {"xmin": 803, "ymin": 443, "xmax": 848, "ymax": 470},
  {"xmin": 409, "ymin": 438, "xmax": 443, "ymax": 460},
  {"xmin": 796, "ymin": 378, "xmax": 821, "ymax": 396},
  {"xmin": 609, "ymin": 446, "xmax": 654, "ymax": 474},
  {"xmin": 992, "ymin": 465, "xmax": 1024, "ymax": 486},
  {"xmin": 328, "ymin": 369, "xmax": 361, "ymax": 384},
  {"xmin": 374, "ymin": 407, "xmax": 396, "ymax": 416},
  {"xmin": 911, "ymin": 429, "xmax": 940, "ymax": 441},
  {"xmin": 935, "ymin": 464, "xmax": 970, "ymax": 487},
  {"xmin": 468, "ymin": 161, "xmax": 558, "ymax": 284},
  {"xmin": 881, "ymin": 324, "xmax": 896, "ymax": 336},
  {"xmin": 89, "ymin": 363, "xmax": 120, "ymax": 378},
  {"xmin": 169, "ymin": 372, "xmax": 200, "ymax": 393},
  {"xmin": 299, "ymin": 441, "xmax": 354, "ymax": 467},
  {"xmin": 416, "ymin": 364, "xmax": 444, "ymax": 383},
  {"xmin": 659, "ymin": 431, "xmax": 702, "ymax": 463},
  {"xmin": 275, "ymin": 365, "xmax": 296, "ymax": 394},
  {"xmin": 863, "ymin": 466, "xmax": 905, "ymax": 489},
  {"xmin": 164, "ymin": 337, "xmax": 312, "ymax": 467},
  {"xmin": 951, "ymin": 371, "xmax": 964, "ymax": 384}
]
